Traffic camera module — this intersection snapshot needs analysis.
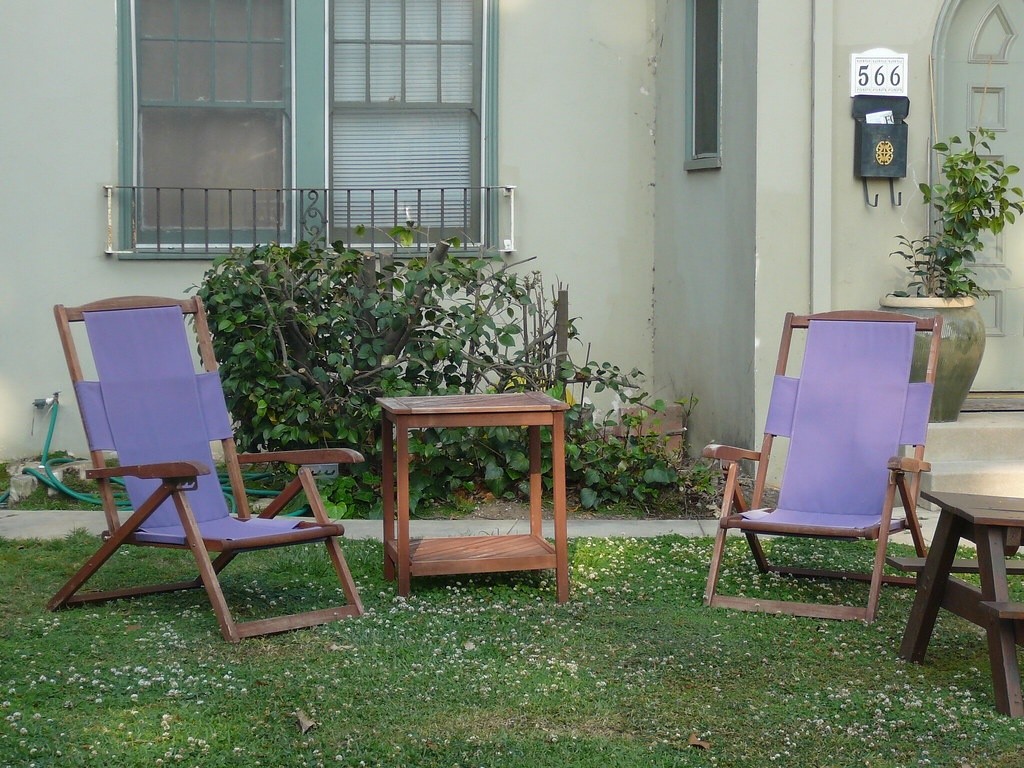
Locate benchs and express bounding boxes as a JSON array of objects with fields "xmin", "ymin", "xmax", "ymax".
[
  {"xmin": 978, "ymin": 601, "xmax": 1024, "ymax": 717},
  {"xmin": 885, "ymin": 557, "xmax": 1024, "ymax": 667}
]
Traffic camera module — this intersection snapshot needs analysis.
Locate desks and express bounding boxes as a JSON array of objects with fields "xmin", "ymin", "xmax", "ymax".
[{"xmin": 899, "ymin": 490, "xmax": 1024, "ymax": 717}]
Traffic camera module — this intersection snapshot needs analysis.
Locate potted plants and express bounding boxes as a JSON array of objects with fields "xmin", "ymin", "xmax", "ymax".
[{"xmin": 879, "ymin": 128, "xmax": 1024, "ymax": 424}]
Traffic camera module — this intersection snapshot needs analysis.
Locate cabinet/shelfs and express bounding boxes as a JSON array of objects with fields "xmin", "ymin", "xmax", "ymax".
[{"xmin": 375, "ymin": 390, "xmax": 572, "ymax": 605}]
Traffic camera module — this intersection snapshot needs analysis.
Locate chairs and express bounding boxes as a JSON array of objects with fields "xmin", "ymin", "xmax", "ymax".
[
  {"xmin": 48, "ymin": 296, "xmax": 365, "ymax": 644},
  {"xmin": 702, "ymin": 309, "xmax": 944, "ymax": 623}
]
